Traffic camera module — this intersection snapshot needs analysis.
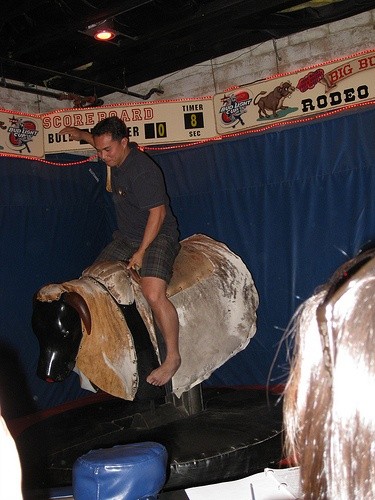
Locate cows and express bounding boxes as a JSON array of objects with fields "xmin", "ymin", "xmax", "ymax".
[
  {"xmin": 267, "ymin": 249, "xmax": 375, "ymax": 499},
  {"xmin": 33, "ymin": 235, "xmax": 260, "ymax": 414}
]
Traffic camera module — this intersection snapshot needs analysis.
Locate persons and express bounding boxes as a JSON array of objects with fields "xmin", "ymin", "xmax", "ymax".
[
  {"xmin": 274, "ymin": 248, "xmax": 375, "ymax": 500},
  {"xmin": 57, "ymin": 115, "xmax": 182, "ymax": 387}
]
